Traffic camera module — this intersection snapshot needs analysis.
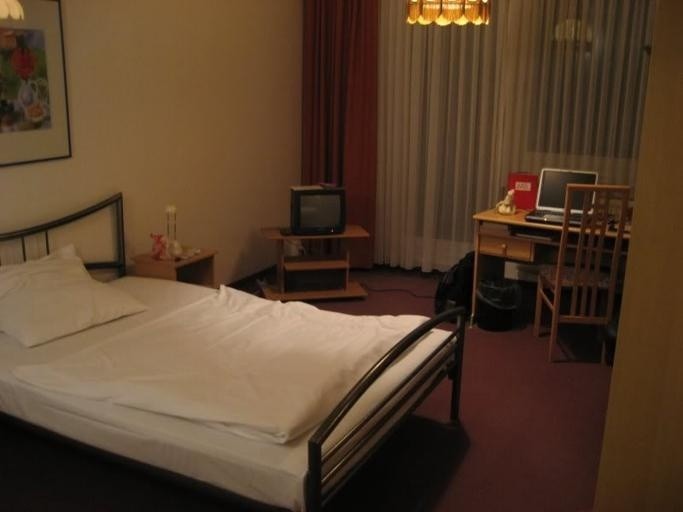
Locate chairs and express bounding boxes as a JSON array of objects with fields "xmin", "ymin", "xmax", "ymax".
[{"xmin": 531, "ymin": 180, "xmax": 634, "ymax": 367}]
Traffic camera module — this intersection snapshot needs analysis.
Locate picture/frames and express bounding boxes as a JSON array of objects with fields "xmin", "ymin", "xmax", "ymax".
[{"xmin": 0, "ymin": 1, "xmax": 73, "ymax": 172}]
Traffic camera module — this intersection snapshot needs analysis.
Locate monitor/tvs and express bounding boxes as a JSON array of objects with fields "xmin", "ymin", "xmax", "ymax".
[{"xmin": 290, "ymin": 186, "xmax": 346, "ymax": 235}]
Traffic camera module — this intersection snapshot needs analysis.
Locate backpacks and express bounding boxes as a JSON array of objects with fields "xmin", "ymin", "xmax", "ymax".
[{"xmin": 434, "ymin": 250, "xmax": 475, "ymax": 324}]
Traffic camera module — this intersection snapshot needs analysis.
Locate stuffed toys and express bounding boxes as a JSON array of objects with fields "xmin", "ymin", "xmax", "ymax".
[{"xmin": 146, "ymin": 232, "xmax": 166, "ymax": 260}]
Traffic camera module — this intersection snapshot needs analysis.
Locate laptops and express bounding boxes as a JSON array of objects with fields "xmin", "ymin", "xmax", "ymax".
[{"xmin": 525, "ymin": 168, "xmax": 598, "ymax": 227}]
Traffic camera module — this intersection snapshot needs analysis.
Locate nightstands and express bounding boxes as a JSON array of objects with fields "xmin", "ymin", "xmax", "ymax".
[{"xmin": 132, "ymin": 239, "xmax": 219, "ymax": 288}]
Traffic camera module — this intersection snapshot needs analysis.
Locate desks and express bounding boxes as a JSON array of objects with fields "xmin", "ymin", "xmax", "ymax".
[{"xmin": 468, "ymin": 201, "xmax": 636, "ymax": 339}]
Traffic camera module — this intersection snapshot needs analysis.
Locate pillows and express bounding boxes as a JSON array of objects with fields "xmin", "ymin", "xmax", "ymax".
[{"xmin": 1, "ymin": 236, "xmax": 151, "ymax": 351}]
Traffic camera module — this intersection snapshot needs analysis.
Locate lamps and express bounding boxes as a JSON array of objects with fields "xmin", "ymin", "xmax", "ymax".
[{"xmin": 402, "ymin": 0, "xmax": 494, "ymax": 28}]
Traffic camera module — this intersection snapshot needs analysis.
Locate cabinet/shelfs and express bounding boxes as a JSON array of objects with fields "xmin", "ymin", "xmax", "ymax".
[{"xmin": 255, "ymin": 222, "xmax": 374, "ymax": 306}]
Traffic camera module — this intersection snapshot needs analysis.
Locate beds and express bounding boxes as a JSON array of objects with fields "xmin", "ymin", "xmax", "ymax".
[{"xmin": 1, "ymin": 192, "xmax": 473, "ymax": 510}]
